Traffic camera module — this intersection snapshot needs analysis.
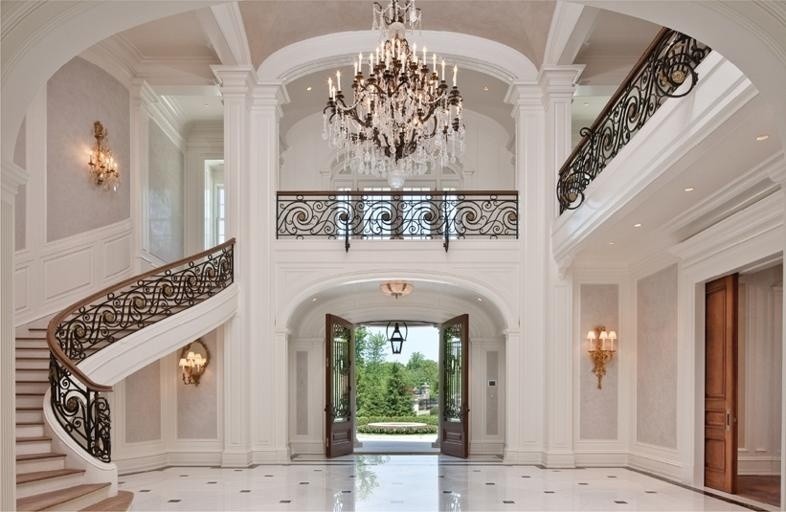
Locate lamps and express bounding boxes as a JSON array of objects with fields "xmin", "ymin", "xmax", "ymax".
[
  {"xmin": 586, "ymin": 325, "xmax": 617, "ymax": 390},
  {"xmin": 378, "ymin": 283, "xmax": 415, "ymax": 300},
  {"xmin": 322, "ymin": 0, "xmax": 468, "ymax": 188},
  {"xmin": 88, "ymin": 120, "xmax": 121, "ymax": 192},
  {"xmin": 386, "ymin": 320, "xmax": 408, "ymax": 353},
  {"xmin": 179, "ymin": 339, "xmax": 211, "ymax": 386}
]
